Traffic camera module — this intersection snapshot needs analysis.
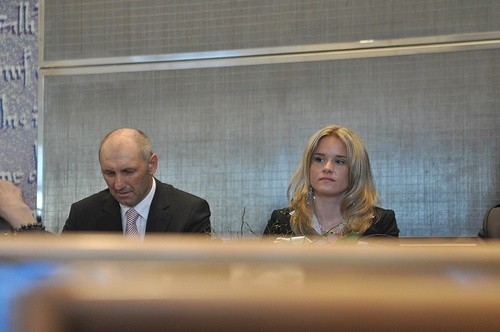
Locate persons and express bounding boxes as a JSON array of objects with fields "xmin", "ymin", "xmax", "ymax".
[
  {"xmin": 256, "ymin": 126, "xmax": 400, "ymax": 241},
  {"xmin": 475, "ymin": 198, "xmax": 499, "ymax": 238},
  {"xmin": 0, "ymin": 175, "xmax": 53, "ymax": 232},
  {"xmin": 56, "ymin": 128, "xmax": 211, "ymax": 235}
]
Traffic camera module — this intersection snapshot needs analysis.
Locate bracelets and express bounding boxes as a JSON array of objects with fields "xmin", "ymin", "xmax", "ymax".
[{"xmin": 7, "ymin": 223, "xmax": 46, "ymax": 233}]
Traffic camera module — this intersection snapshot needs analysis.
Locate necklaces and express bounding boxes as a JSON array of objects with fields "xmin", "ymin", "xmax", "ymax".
[{"xmin": 308, "ymin": 187, "xmax": 355, "ymax": 235}]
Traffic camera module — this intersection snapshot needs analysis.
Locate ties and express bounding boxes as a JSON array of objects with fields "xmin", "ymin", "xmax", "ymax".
[{"xmin": 125, "ymin": 208, "xmax": 140, "ymax": 235}]
